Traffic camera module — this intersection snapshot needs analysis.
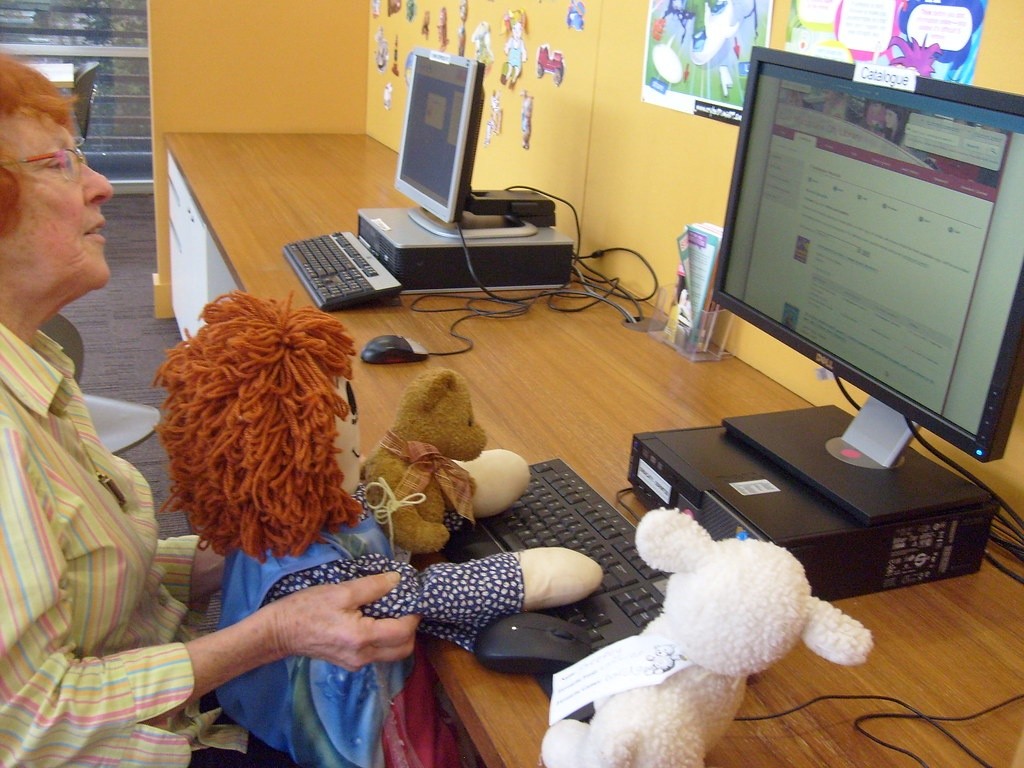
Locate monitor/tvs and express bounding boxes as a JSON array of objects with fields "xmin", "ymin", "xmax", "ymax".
[
  {"xmin": 713, "ymin": 45, "xmax": 1024, "ymax": 524},
  {"xmin": 394, "ymin": 46, "xmax": 556, "ymax": 238}
]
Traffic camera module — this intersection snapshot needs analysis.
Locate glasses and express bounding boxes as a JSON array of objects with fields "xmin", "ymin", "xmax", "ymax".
[{"xmin": 0, "ymin": 147, "xmax": 87, "ymax": 183}]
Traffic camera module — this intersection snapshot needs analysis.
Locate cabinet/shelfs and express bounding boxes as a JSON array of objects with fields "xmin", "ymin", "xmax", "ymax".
[{"xmin": 167, "ymin": 149, "xmax": 240, "ymax": 348}]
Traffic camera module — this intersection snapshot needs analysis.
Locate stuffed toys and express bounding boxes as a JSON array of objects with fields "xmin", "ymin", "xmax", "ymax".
[
  {"xmin": 543, "ymin": 508, "xmax": 872, "ymax": 768},
  {"xmin": 363, "ymin": 368, "xmax": 486, "ymax": 552}
]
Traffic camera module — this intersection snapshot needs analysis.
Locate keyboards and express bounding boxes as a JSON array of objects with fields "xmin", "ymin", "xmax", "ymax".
[
  {"xmin": 441, "ymin": 458, "xmax": 676, "ymax": 723},
  {"xmin": 283, "ymin": 231, "xmax": 402, "ymax": 314}
]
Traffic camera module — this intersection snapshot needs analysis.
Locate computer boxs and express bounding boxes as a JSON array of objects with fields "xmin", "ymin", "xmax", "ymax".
[
  {"xmin": 356, "ymin": 207, "xmax": 575, "ymax": 294},
  {"xmin": 626, "ymin": 424, "xmax": 1001, "ymax": 601}
]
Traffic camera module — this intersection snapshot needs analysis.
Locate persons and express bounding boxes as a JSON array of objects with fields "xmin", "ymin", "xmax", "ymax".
[
  {"xmin": 153, "ymin": 291, "xmax": 604, "ymax": 768},
  {"xmin": 0, "ymin": 49, "xmax": 419, "ymax": 768},
  {"xmin": 883, "ymin": 105, "xmax": 904, "ymax": 145}
]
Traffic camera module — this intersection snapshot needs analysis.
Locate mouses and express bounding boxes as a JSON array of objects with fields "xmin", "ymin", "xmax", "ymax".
[
  {"xmin": 360, "ymin": 334, "xmax": 428, "ymax": 364},
  {"xmin": 472, "ymin": 613, "xmax": 592, "ymax": 675}
]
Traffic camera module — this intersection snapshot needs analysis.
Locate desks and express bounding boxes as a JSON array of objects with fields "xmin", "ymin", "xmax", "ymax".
[
  {"xmin": 21, "ymin": 63, "xmax": 76, "ymax": 89},
  {"xmin": 163, "ymin": 129, "xmax": 1024, "ymax": 768}
]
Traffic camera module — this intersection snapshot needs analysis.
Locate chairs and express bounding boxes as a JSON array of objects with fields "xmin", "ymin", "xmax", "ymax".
[{"xmin": 71, "ymin": 61, "xmax": 101, "ymax": 151}]
